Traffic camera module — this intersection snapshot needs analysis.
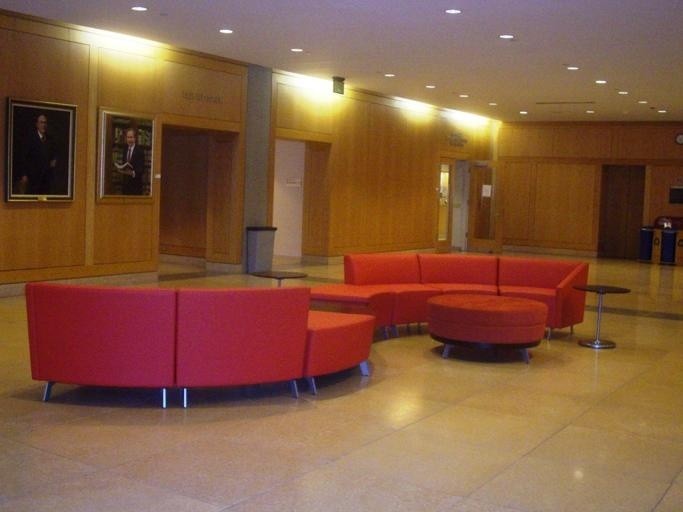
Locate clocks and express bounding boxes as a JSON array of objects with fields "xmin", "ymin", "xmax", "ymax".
[{"xmin": 675, "ymin": 134, "xmax": 682, "ymax": 145}]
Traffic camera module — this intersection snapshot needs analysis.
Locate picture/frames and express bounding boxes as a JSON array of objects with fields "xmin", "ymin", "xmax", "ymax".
[
  {"xmin": 96, "ymin": 106, "xmax": 156, "ymax": 204},
  {"xmin": 6, "ymin": 96, "xmax": 78, "ymax": 204}
]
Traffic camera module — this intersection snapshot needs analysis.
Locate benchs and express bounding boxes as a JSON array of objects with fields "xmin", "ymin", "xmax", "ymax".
[
  {"xmin": 26, "ymin": 282, "xmax": 376, "ymax": 407},
  {"xmin": 310, "ymin": 254, "xmax": 589, "ymax": 341}
]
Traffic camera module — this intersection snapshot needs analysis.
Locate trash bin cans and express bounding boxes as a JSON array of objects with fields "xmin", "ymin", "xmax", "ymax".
[{"xmin": 246, "ymin": 226, "xmax": 278, "ymax": 274}]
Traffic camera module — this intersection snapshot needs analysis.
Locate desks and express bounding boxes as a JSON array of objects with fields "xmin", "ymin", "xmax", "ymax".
[
  {"xmin": 248, "ymin": 269, "xmax": 307, "ymax": 285},
  {"xmin": 575, "ymin": 283, "xmax": 630, "ymax": 349}
]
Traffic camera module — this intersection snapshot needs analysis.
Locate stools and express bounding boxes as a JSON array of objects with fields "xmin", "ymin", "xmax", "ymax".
[{"xmin": 426, "ymin": 294, "xmax": 549, "ymax": 363}]
